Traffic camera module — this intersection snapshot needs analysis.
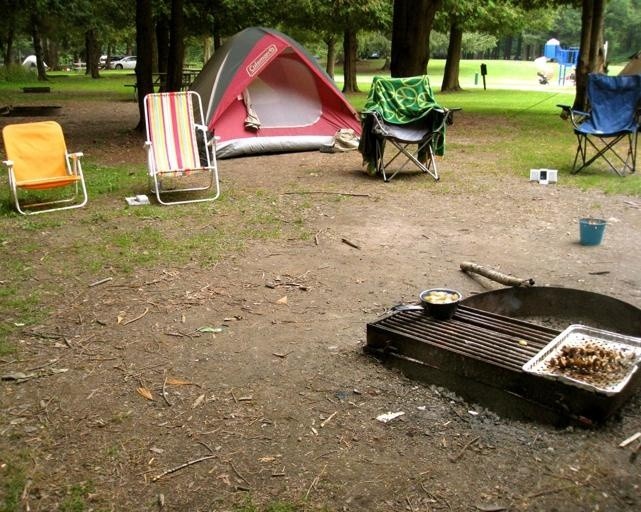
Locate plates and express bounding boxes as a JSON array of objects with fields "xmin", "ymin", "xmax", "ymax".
[{"xmin": 525, "ymin": 322, "xmax": 641, "ymax": 394}]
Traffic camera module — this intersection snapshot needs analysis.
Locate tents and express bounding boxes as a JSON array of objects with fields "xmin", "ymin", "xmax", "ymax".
[
  {"xmin": 183, "ymin": 26, "xmax": 363, "ymax": 161},
  {"xmin": 21, "ymin": 54, "xmax": 49, "ymax": 73}
]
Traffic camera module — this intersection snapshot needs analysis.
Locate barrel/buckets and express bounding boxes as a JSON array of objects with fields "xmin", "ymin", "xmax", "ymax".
[{"xmin": 579, "ymin": 208, "xmax": 608, "ymax": 245}]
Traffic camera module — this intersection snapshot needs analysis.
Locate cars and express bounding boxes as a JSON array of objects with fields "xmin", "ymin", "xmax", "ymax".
[{"xmin": 108, "ymin": 55, "xmax": 137, "ymax": 70}]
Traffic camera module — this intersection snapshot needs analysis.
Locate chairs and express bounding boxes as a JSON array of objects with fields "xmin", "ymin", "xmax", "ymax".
[
  {"xmin": 144, "ymin": 91, "xmax": 220, "ymax": 205},
  {"xmin": 358, "ymin": 75, "xmax": 462, "ymax": 182},
  {"xmin": 557, "ymin": 73, "xmax": 641, "ymax": 177},
  {"xmin": 2, "ymin": 121, "xmax": 88, "ymax": 215}
]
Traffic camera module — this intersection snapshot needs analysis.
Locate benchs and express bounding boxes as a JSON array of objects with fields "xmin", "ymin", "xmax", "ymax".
[
  {"xmin": 124, "ymin": 82, "xmax": 192, "ymax": 102},
  {"xmin": 127, "ymin": 73, "xmax": 199, "ymax": 83},
  {"xmin": 183, "ymin": 64, "xmax": 196, "ymax": 68},
  {"xmin": 182, "ymin": 68, "xmax": 201, "ymax": 71}
]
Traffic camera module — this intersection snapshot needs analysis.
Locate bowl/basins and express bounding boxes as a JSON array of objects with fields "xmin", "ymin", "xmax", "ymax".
[{"xmin": 420, "ymin": 288, "xmax": 462, "ymax": 314}]
